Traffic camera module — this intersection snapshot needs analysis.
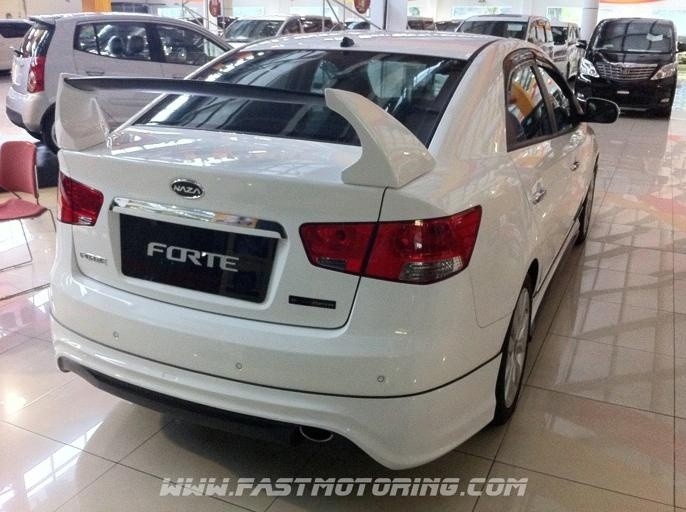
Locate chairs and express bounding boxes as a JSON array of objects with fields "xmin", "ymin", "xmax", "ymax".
[
  {"xmin": 0, "ymin": 140, "xmax": 56, "ymax": 301},
  {"xmin": 109, "ymin": 35, "xmax": 148, "ymax": 60}
]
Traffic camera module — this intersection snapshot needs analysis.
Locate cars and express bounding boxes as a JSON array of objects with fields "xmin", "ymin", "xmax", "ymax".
[
  {"xmin": 37, "ymin": 31, "xmax": 603, "ymax": 472},
  {"xmin": 1, "ymin": 10, "xmax": 686, "ymax": 159}
]
